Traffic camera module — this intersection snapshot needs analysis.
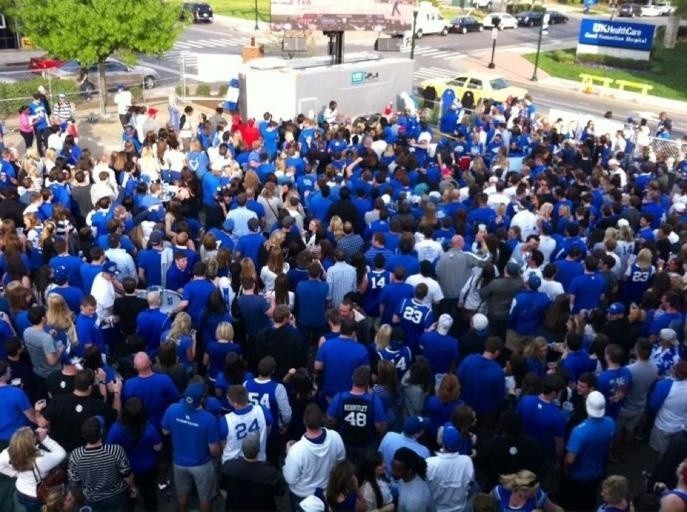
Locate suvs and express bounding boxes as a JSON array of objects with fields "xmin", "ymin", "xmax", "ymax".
[{"xmin": 179, "ymin": 2, "xmax": 214, "ymax": 23}]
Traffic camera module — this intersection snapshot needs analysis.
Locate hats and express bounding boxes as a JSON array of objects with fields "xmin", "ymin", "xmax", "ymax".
[
  {"xmin": 101, "ymin": 261, "xmax": 121, "ymax": 275},
  {"xmin": 606, "ymin": 302, "xmax": 625, "ymax": 314},
  {"xmin": 441, "ymin": 421, "xmax": 462, "ymax": 450},
  {"xmin": 529, "ymin": 272, "xmax": 541, "ymax": 288},
  {"xmin": 298, "ymin": 493, "xmax": 325, "ymax": 512},
  {"xmin": 674, "ymin": 201, "xmax": 687, "ymax": 213},
  {"xmin": 472, "ymin": 313, "xmax": 490, "ymax": 332},
  {"xmin": 436, "ymin": 313, "xmax": 453, "ymax": 336},
  {"xmin": 659, "ymin": 328, "xmax": 680, "ymax": 347},
  {"xmin": 80, "ymin": 415, "xmax": 107, "ymax": 441},
  {"xmin": 402, "ymin": 415, "xmax": 431, "ymax": 435},
  {"xmin": 585, "ymin": 390, "xmax": 606, "ymax": 419},
  {"xmin": 183, "ymin": 381, "xmax": 204, "ymax": 409}
]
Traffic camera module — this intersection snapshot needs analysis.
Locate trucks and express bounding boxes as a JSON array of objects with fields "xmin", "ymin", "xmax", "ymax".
[{"xmin": 414, "ymin": 0, "xmax": 451, "ymax": 38}]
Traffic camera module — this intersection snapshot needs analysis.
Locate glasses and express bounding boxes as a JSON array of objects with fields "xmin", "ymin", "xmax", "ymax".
[{"xmin": 519, "ymin": 472, "xmax": 540, "ymax": 489}]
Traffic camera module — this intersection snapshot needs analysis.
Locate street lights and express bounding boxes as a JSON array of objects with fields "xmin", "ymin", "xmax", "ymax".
[
  {"xmin": 529, "ymin": 0, "xmax": 554, "ymax": 82},
  {"xmin": 410, "ymin": 5, "xmax": 419, "ymax": 59}
]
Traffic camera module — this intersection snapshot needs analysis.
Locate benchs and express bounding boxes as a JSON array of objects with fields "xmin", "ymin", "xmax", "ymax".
[
  {"xmin": 615, "ymin": 78, "xmax": 653, "ymax": 102},
  {"xmin": 578, "ymin": 72, "xmax": 614, "ymax": 97}
]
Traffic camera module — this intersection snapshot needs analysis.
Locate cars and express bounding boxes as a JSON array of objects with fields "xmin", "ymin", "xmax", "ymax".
[
  {"xmin": 618, "ymin": 0, "xmax": 678, "ymax": 18},
  {"xmin": 416, "ymin": 72, "xmax": 530, "ymax": 109},
  {"xmin": 40, "ymin": 56, "xmax": 160, "ymax": 97},
  {"xmin": 26, "ymin": 48, "xmax": 67, "ymax": 76},
  {"xmin": 449, "ymin": 7, "xmax": 571, "ymax": 34}
]
